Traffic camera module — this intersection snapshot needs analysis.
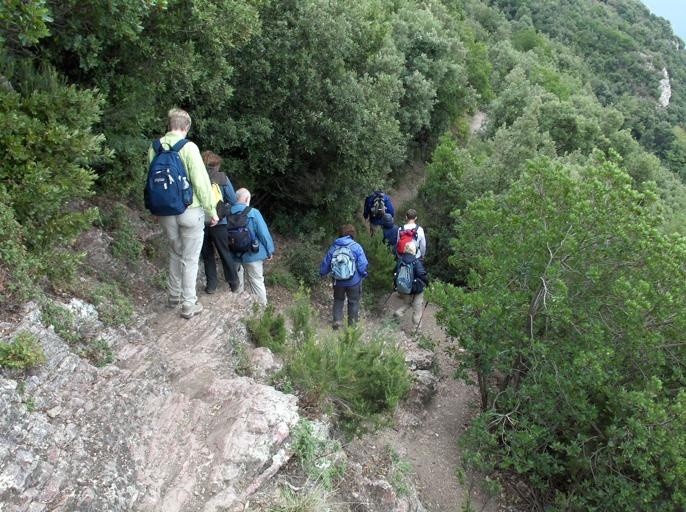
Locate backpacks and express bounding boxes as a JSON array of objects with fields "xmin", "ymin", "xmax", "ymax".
[
  {"xmin": 331, "ymin": 242, "xmax": 356, "ymax": 280},
  {"xmin": 144, "ymin": 140, "xmax": 192, "ymax": 215},
  {"xmin": 370, "ymin": 194, "xmax": 386, "ymax": 219},
  {"xmin": 227, "ymin": 207, "xmax": 259, "ymax": 253},
  {"xmin": 396, "ymin": 225, "xmax": 421, "ymax": 255},
  {"xmin": 397, "ymin": 260, "xmax": 419, "ymax": 294},
  {"xmin": 207, "ymin": 171, "xmax": 229, "ymax": 221}
]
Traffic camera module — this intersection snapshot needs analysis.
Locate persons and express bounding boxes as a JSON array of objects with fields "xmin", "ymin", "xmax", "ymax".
[
  {"xmin": 318, "ymin": 223, "xmax": 368, "ymax": 331},
  {"xmin": 146, "ymin": 106, "xmax": 218, "ymax": 318},
  {"xmin": 362, "ymin": 188, "xmax": 394, "ymax": 236},
  {"xmin": 391, "ymin": 241, "xmax": 434, "ymax": 333},
  {"xmin": 199, "ymin": 149, "xmax": 241, "ymax": 295},
  {"xmin": 395, "ymin": 208, "xmax": 426, "ymax": 265},
  {"xmin": 228, "ymin": 188, "xmax": 275, "ymax": 299},
  {"xmin": 380, "ymin": 213, "xmax": 399, "ymax": 249}
]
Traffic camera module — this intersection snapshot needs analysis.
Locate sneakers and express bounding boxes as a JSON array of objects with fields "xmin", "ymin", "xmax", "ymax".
[
  {"xmin": 181, "ymin": 302, "xmax": 204, "ymax": 318},
  {"xmin": 169, "ymin": 295, "xmax": 181, "ymax": 304}
]
